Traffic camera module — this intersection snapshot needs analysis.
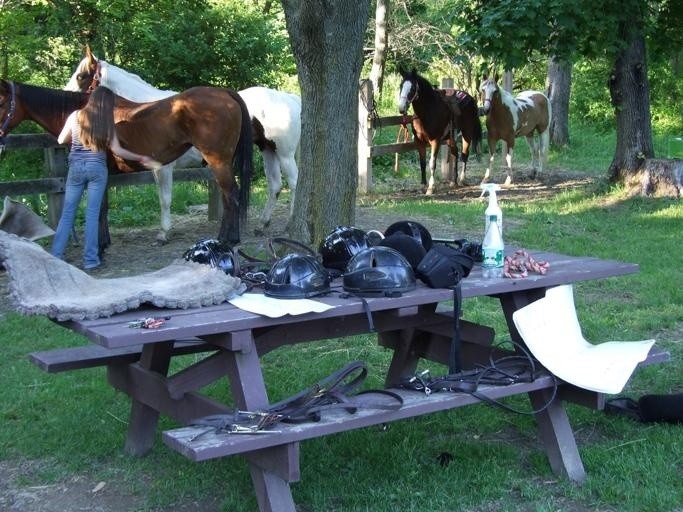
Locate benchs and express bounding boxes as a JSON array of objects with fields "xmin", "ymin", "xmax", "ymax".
[
  {"xmin": 159, "ymin": 337, "xmax": 674, "ymax": 511},
  {"xmin": 25, "ymin": 301, "xmax": 464, "ymax": 462}
]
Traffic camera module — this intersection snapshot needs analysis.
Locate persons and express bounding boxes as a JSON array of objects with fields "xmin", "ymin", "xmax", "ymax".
[{"xmin": 48, "ymin": 86, "xmax": 161, "ymax": 270}]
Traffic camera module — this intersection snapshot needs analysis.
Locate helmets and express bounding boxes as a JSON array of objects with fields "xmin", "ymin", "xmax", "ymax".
[
  {"xmin": 343, "ymin": 245, "xmax": 418, "ymax": 298},
  {"xmin": 181, "ymin": 238, "xmax": 242, "ymax": 278},
  {"xmin": 318, "ymin": 226, "xmax": 373, "ymax": 270},
  {"xmin": 375, "ymin": 219, "xmax": 433, "ymax": 270},
  {"xmin": 263, "ymin": 253, "xmax": 329, "ymax": 299}
]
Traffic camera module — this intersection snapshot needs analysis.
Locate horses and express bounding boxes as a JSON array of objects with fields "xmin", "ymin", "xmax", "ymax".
[
  {"xmin": 63, "ymin": 44, "xmax": 301, "ymax": 246},
  {"xmin": 479, "ymin": 70, "xmax": 553, "ymax": 187},
  {"xmin": 398, "ymin": 63, "xmax": 482, "ymax": 197},
  {"xmin": 0, "ymin": 76, "xmax": 253, "ymax": 255}
]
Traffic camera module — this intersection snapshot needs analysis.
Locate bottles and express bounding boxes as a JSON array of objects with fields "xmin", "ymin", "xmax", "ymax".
[
  {"xmin": 482, "ymin": 215, "xmax": 504, "ymax": 278},
  {"xmin": 484, "ymin": 188, "xmax": 503, "ymax": 239}
]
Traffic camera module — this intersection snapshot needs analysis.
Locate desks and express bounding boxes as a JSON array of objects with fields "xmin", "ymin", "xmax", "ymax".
[{"xmin": 45, "ymin": 238, "xmax": 637, "ymax": 512}]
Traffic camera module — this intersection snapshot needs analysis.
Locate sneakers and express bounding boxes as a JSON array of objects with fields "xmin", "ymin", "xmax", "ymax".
[{"xmin": 83, "ymin": 264, "xmax": 113, "ymax": 278}]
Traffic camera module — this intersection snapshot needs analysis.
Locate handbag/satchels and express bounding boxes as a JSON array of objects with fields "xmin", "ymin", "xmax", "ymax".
[{"xmin": 415, "ymin": 244, "xmax": 475, "ymax": 289}]
